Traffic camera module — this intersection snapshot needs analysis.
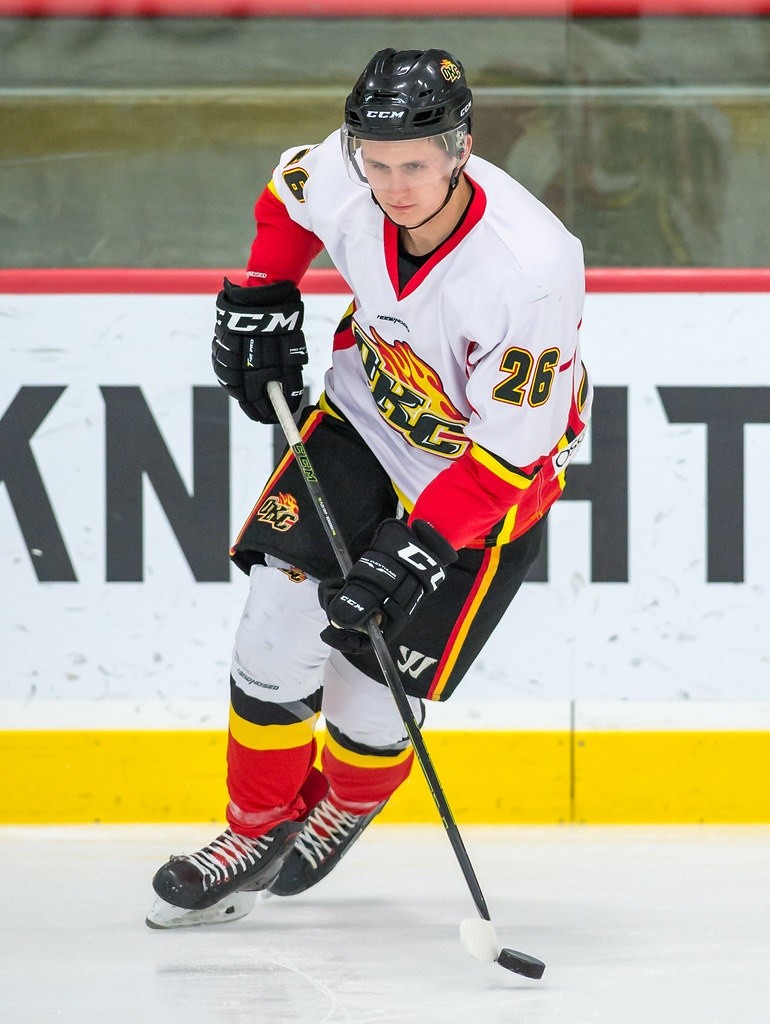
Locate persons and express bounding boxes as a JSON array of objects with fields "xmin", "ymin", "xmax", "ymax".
[
  {"xmin": 147, "ymin": 47, "xmax": 595, "ymax": 929},
  {"xmin": 518, "ymin": 2, "xmax": 737, "ymax": 268}
]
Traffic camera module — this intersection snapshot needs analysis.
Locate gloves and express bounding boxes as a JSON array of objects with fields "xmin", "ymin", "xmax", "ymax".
[
  {"xmin": 211, "ymin": 276, "xmax": 308, "ymax": 425},
  {"xmin": 318, "ymin": 520, "xmax": 459, "ymax": 654}
]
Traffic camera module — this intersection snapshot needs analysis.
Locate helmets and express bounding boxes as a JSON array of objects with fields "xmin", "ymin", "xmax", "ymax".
[{"xmin": 344, "ymin": 48, "xmax": 473, "ymax": 158}]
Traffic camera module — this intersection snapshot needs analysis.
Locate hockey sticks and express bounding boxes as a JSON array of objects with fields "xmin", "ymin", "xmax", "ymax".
[{"xmin": 268, "ymin": 381, "xmax": 510, "ymax": 961}]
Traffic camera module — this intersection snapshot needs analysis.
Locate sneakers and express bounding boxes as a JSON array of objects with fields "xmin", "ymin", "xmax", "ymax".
[
  {"xmin": 267, "ymin": 787, "xmax": 391, "ymax": 896},
  {"xmin": 146, "ymin": 819, "xmax": 309, "ymax": 930}
]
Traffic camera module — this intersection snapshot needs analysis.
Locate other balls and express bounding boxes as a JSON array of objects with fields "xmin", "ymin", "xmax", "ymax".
[{"xmin": 497, "ymin": 948, "xmax": 547, "ymax": 981}]
